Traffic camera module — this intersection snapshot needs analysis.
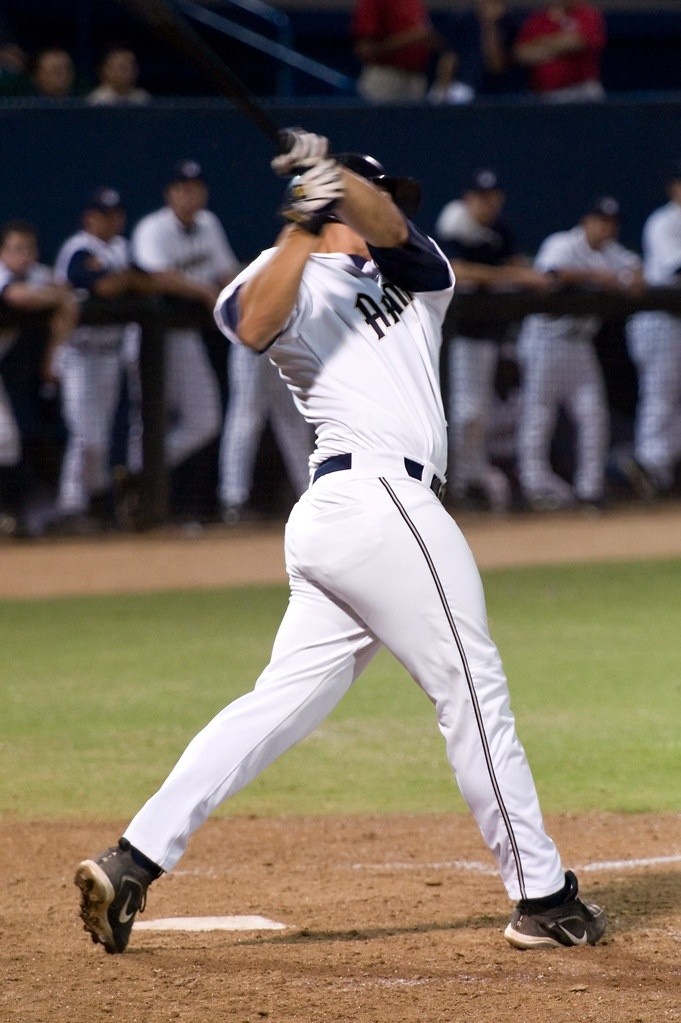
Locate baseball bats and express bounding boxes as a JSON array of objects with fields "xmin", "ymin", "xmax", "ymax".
[{"xmin": 128, "ymin": 0, "xmax": 295, "ymax": 155}]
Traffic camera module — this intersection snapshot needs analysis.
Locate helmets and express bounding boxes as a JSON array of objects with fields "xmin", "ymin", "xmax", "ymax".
[{"xmin": 300, "ymin": 152, "xmax": 391, "ymax": 233}]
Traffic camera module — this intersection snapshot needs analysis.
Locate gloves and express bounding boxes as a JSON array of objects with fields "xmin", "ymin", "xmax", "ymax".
[
  {"xmin": 261, "ymin": 122, "xmax": 329, "ymax": 172},
  {"xmin": 279, "ymin": 158, "xmax": 346, "ymax": 236}
]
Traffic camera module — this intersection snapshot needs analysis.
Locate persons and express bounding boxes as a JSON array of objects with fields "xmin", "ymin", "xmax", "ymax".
[
  {"xmin": 515, "ymin": 196, "xmax": 643, "ymax": 504},
  {"xmin": 76, "ymin": 133, "xmax": 610, "ymax": 946},
  {"xmin": 435, "ymin": 173, "xmax": 548, "ymax": 504},
  {"xmin": 351, "ymin": 0, "xmax": 436, "ymax": 103},
  {"xmin": 131, "ymin": 150, "xmax": 241, "ymax": 516},
  {"xmin": 0, "ymin": 223, "xmax": 77, "ymax": 528},
  {"xmin": 216, "ymin": 341, "xmax": 315, "ymax": 517},
  {"xmin": 624, "ymin": 178, "xmax": 681, "ymax": 490},
  {"xmin": 517, "ymin": 0, "xmax": 602, "ymax": 103},
  {"xmin": 34, "ymin": 52, "xmax": 74, "ymax": 95},
  {"xmin": 0, "ymin": 45, "xmax": 32, "ymax": 95},
  {"xmin": 84, "ymin": 50, "xmax": 152, "ymax": 105},
  {"xmin": 51, "ymin": 188, "xmax": 154, "ymax": 532},
  {"xmin": 427, "ymin": 0, "xmax": 525, "ymax": 103}
]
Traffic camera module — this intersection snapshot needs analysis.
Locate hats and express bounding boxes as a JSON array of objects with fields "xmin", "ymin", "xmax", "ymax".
[
  {"xmin": 466, "ymin": 162, "xmax": 501, "ymax": 190},
  {"xmin": 170, "ymin": 158, "xmax": 205, "ymax": 184},
  {"xmin": 85, "ymin": 184, "xmax": 126, "ymax": 212},
  {"xmin": 588, "ymin": 190, "xmax": 622, "ymax": 219}
]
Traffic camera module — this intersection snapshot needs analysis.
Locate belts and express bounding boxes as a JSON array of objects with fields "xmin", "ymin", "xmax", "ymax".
[{"xmin": 314, "ymin": 451, "xmax": 446, "ymax": 501}]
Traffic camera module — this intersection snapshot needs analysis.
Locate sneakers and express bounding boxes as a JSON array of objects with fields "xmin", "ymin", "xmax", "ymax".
[
  {"xmin": 74, "ymin": 845, "xmax": 153, "ymax": 954},
  {"xmin": 505, "ymin": 871, "xmax": 607, "ymax": 951}
]
